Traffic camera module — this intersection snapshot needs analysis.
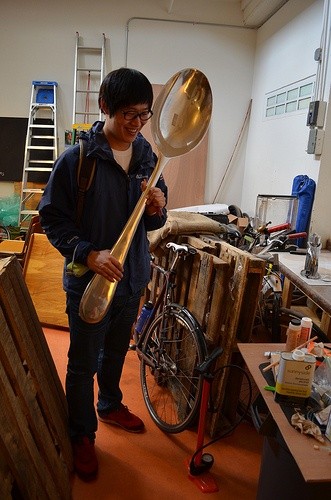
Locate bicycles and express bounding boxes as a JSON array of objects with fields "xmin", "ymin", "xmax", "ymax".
[
  {"xmin": 127, "ymin": 242, "xmax": 207, "ymax": 434},
  {"xmin": 220, "ymin": 221, "xmax": 330, "ymax": 425}
]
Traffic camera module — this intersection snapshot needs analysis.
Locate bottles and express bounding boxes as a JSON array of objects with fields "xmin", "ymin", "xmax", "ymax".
[
  {"xmin": 273, "ymin": 350, "xmax": 316, "ymax": 408},
  {"xmin": 298, "ymin": 316, "xmax": 314, "ymax": 349},
  {"xmin": 284, "ymin": 318, "xmax": 302, "ymax": 352},
  {"xmin": 135, "ymin": 300, "xmax": 154, "ymax": 335}
]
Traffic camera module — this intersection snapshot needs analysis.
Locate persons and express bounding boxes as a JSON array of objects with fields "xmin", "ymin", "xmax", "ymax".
[{"xmin": 38, "ymin": 68, "xmax": 169, "ymax": 482}]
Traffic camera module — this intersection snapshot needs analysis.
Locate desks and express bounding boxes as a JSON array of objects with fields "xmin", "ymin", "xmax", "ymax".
[
  {"xmin": 266, "ymin": 252, "xmax": 331, "ymax": 342},
  {"xmin": 236, "ymin": 342, "xmax": 331, "ymax": 500}
]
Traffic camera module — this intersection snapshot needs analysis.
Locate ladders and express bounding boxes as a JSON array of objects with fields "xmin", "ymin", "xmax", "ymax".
[
  {"xmin": 16, "ymin": 81, "xmax": 59, "ymax": 225},
  {"xmin": 70, "ymin": 31, "xmax": 106, "ymax": 146}
]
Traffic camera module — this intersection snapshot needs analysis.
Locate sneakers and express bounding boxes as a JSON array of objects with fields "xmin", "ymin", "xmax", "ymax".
[
  {"xmin": 73, "ymin": 436, "xmax": 99, "ymax": 473},
  {"xmin": 97, "ymin": 403, "xmax": 144, "ymax": 433}
]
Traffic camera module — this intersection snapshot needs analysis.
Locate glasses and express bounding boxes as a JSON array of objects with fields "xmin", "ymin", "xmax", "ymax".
[{"xmin": 118, "ymin": 105, "xmax": 154, "ymax": 122}]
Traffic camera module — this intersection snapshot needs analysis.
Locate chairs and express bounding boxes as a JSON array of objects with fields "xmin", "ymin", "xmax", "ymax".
[{"xmin": 0, "ymin": 215, "xmax": 43, "ymax": 267}]
